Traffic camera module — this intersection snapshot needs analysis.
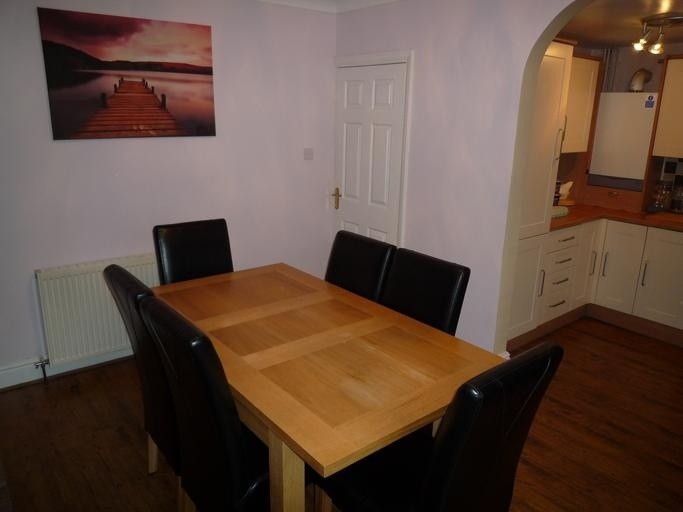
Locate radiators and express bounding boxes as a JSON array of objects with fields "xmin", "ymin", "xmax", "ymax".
[{"xmin": 34, "ymin": 255, "xmax": 159, "ymax": 366}]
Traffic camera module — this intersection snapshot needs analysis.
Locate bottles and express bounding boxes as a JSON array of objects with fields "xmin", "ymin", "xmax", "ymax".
[
  {"xmin": 651, "ymin": 180, "xmax": 673, "ymax": 209},
  {"xmin": 552, "ymin": 194, "xmax": 560, "ymax": 207},
  {"xmin": 554, "ymin": 181, "xmax": 560, "ymax": 193},
  {"xmin": 669, "ymin": 175, "xmax": 683, "ymax": 214}
]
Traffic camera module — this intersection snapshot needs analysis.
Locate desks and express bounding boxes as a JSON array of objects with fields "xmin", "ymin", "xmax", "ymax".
[{"xmin": 149, "ymin": 261, "xmax": 509, "ymax": 512}]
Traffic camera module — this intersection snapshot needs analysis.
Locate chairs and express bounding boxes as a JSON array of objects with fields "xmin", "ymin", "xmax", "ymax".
[
  {"xmin": 139, "ymin": 299, "xmax": 316, "ymax": 512},
  {"xmin": 377, "ymin": 248, "xmax": 472, "ymax": 334},
  {"xmin": 311, "ymin": 344, "xmax": 564, "ymax": 511},
  {"xmin": 154, "ymin": 217, "xmax": 235, "ymax": 287},
  {"xmin": 103, "ymin": 261, "xmax": 186, "ymax": 512},
  {"xmin": 325, "ymin": 230, "xmax": 396, "ymax": 303}
]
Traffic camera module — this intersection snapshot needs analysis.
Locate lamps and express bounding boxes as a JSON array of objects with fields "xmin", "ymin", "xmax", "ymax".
[{"xmin": 634, "ymin": 13, "xmax": 683, "ymax": 56}]
